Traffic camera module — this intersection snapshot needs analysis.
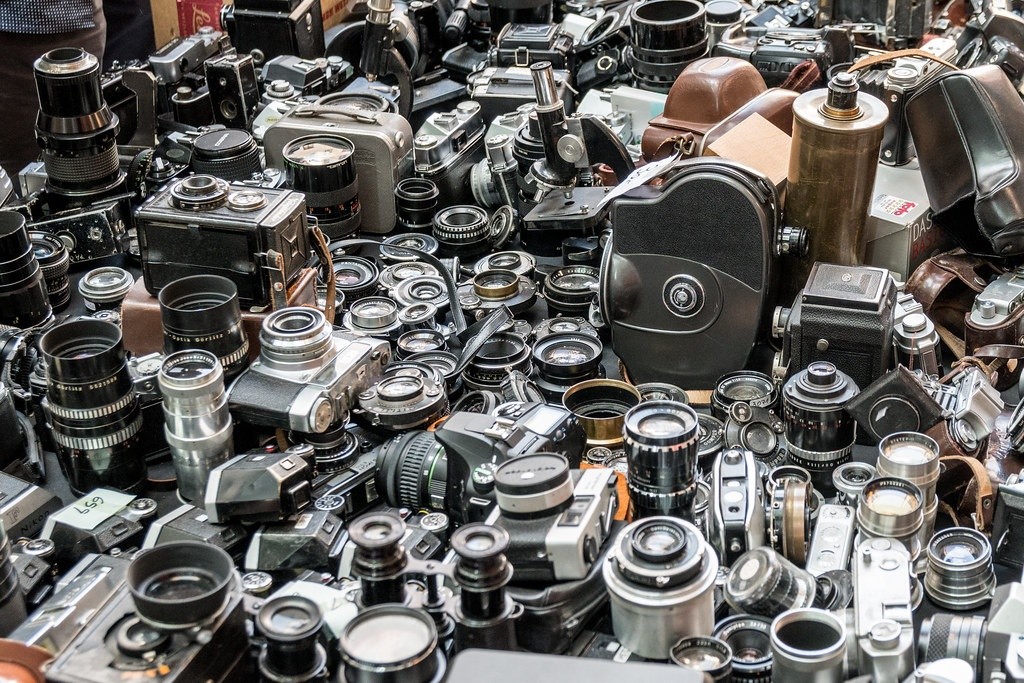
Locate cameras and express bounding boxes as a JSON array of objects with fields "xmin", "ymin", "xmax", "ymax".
[{"xmin": 0, "ymin": 0, "xmax": 1024, "ymax": 683}]
